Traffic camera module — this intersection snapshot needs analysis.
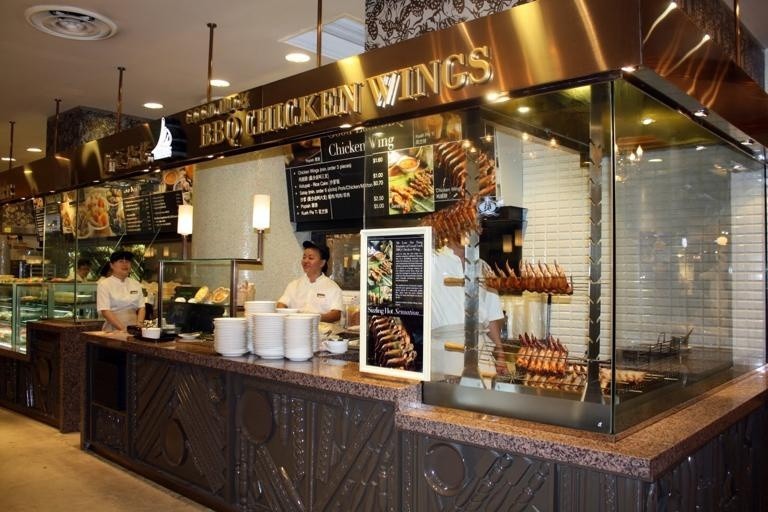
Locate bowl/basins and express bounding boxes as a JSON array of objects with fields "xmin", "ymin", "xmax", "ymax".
[{"xmin": 396, "ymin": 156, "xmax": 420, "ymax": 174}]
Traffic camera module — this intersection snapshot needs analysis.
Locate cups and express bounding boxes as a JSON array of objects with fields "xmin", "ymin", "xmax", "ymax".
[{"xmin": 322, "ymin": 339, "xmax": 349, "ymax": 355}]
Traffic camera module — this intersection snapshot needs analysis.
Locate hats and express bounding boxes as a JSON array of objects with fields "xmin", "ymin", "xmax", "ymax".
[{"xmin": 302, "ymin": 241, "xmax": 329, "ymax": 260}]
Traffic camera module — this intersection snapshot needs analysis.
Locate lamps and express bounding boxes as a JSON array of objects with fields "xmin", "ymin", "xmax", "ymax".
[
  {"xmin": 177, "ymin": 203, "xmax": 194, "ymax": 259},
  {"xmin": 252, "ymin": 193, "xmax": 270, "ymax": 262}
]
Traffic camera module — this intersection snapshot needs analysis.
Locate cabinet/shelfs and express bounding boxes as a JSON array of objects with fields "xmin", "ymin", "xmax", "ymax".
[{"xmin": 0, "ymin": 255, "xmax": 263, "ymax": 354}]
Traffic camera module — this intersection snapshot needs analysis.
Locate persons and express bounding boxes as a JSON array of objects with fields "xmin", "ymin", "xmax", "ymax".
[
  {"xmin": 274, "ymin": 240, "xmax": 346, "ymax": 327},
  {"xmin": 96, "ymin": 262, "xmax": 112, "ymax": 281},
  {"xmin": 96, "ymin": 250, "xmax": 146, "ymax": 334},
  {"xmin": 68, "ymin": 259, "xmax": 92, "ymax": 283},
  {"xmin": 431, "ymin": 232, "xmax": 508, "ymax": 379}
]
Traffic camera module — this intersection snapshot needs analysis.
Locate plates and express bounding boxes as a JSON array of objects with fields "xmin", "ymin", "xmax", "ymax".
[
  {"xmin": 72, "ymin": 194, "xmax": 109, "ymax": 239},
  {"xmin": 348, "ymin": 339, "xmax": 360, "ymax": 350},
  {"xmin": 213, "ymin": 301, "xmax": 320, "ymax": 363},
  {"xmin": 178, "ymin": 333, "xmax": 200, "ymax": 340}
]
